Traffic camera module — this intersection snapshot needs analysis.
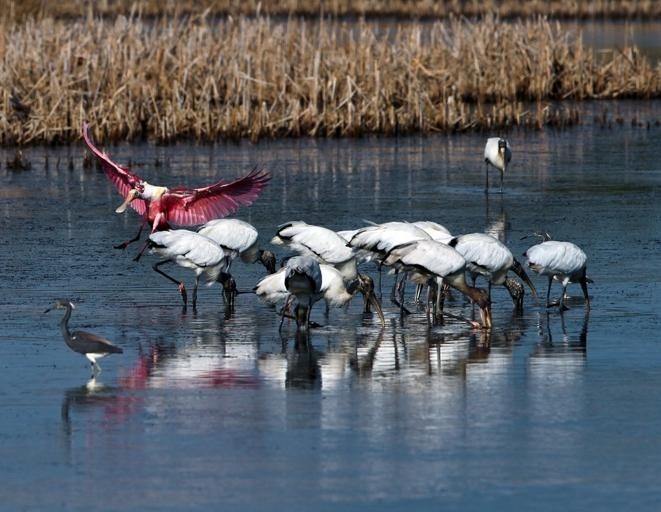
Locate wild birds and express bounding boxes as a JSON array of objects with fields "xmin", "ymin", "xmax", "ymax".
[
  {"xmin": 82, "ymin": 120, "xmax": 272, "ymax": 263},
  {"xmin": 195, "ymin": 218, "xmax": 277, "ymax": 308},
  {"xmin": 252, "ymin": 217, "xmax": 595, "ymax": 333},
  {"xmin": 43, "ymin": 299, "xmax": 124, "ymax": 379},
  {"xmin": 146, "ymin": 229, "xmax": 236, "ymax": 310},
  {"xmin": 483, "ymin": 136, "xmax": 512, "ymax": 191}
]
